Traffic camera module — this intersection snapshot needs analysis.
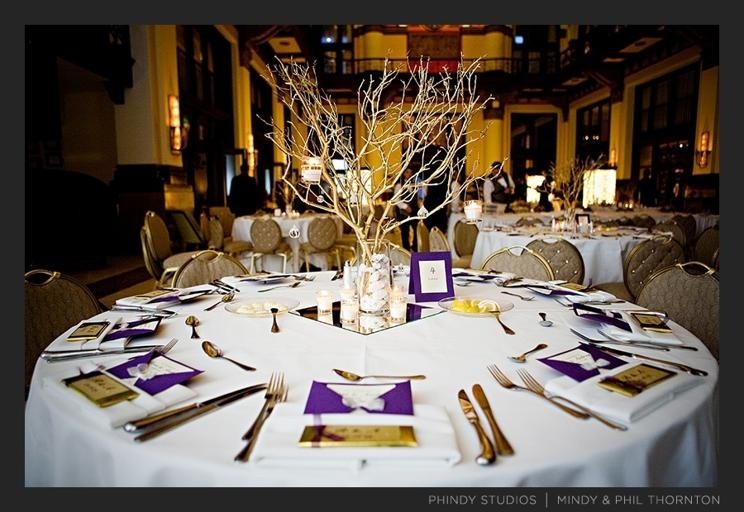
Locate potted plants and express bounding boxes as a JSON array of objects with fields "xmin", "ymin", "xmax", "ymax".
[
  {"xmin": 273, "ymin": 176, "xmax": 303, "ymax": 214},
  {"xmin": 537, "ymin": 153, "xmax": 607, "ymax": 232},
  {"xmin": 253, "ymin": 46, "xmax": 511, "ymax": 317}
]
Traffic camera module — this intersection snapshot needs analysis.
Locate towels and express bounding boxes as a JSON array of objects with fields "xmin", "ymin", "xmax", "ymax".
[
  {"xmin": 597, "ymin": 307, "xmax": 684, "ymax": 348},
  {"xmin": 113, "ymin": 288, "xmax": 182, "ymax": 311},
  {"xmin": 44, "ymin": 360, "xmax": 197, "ymax": 430},
  {"xmin": 220, "ymin": 271, "xmax": 291, "ymax": 289},
  {"xmin": 249, "ymin": 403, "xmax": 462, "ymax": 467},
  {"xmin": 544, "ymin": 356, "xmax": 699, "ymax": 423},
  {"xmin": 532, "ymin": 280, "xmax": 616, "ymax": 305},
  {"xmin": 42, "ymin": 318, "xmax": 144, "ymax": 359}
]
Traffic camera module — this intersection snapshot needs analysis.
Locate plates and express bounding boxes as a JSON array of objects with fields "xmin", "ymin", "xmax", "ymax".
[
  {"xmin": 224, "ymin": 297, "xmax": 300, "ymax": 317},
  {"xmin": 438, "ymin": 295, "xmax": 514, "ymax": 318}
]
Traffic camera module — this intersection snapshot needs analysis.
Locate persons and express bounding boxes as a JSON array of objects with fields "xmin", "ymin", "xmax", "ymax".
[
  {"xmin": 535, "ymin": 164, "xmax": 556, "ymax": 212},
  {"xmin": 483, "ymin": 161, "xmax": 515, "ymax": 206},
  {"xmin": 227, "ymin": 164, "xmax": 259, "ymax": 217},
  {"xmin": 394, "ymin": 169, "xmax": 421, "ymax": 253}
]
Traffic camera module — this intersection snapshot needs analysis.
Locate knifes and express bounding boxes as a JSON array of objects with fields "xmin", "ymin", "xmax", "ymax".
[
  {"xmin": 122, "ymin": 381, "xmax": 267, "ymax": 443},
  {"xmin": 458, "ymin": 384, "xmax": 515, "ymax": 467}
]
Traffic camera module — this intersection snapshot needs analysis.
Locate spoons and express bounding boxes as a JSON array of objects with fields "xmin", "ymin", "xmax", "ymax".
[
  {"xmin": 185, "ymin": 315, "xmax": 200, "ymax": 339},
  {"xmin": 538, "ymin": 311, "xmax": 554, "ymax": 327},
  {"xmin": 490, "ymin": 310, "xmax": 516, "ymax": 336},
  {"xmin": 201, "ymin": 340, "xmax": 258, "ymax": 371},
  {"xmin": 331, "ymin": 367, "xmax": 428, "ymax": 383},
  {"xmin": 508, "ymin": 344, "xmax": 550, "ymax": 363},
  {"xmin": 501, "ymin": 291, "xmax": 537, "ymax": 303},
  {"xmin": 270, "ymin": 308, "xmax": 280, "ymax": 334},
  {"xmin": 257, "ymin": 280, "xmax": 302, "ymax": 292},
  {"xmin": 203, "ymin": 290, "xmax": 236, "ymax": 312}
]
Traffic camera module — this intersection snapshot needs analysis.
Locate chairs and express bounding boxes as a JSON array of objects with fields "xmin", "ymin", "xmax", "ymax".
[
  {"xmin": 592, "ymin": 235, "xmax": 685, "ymax": 305},
  {"xmin": 208, "ymin": 215, "xmax": 253, "ymax": 261},
  {"xmin": 656, "ymin": 219, "xmax": 684, "ymax": 245},
  {"xmin": 171, "ymin": 249, "xmax": 249, "ymax": 290},
  {"xmin": 139, "ymin": 230, "xmax": 170, "ymax": 291},
  {"xmin": 637, "ymin": 261, "xmax": 719, "ymax": 363},
  {"xmin": 527, "ymin": 239, "xmax": 586, "ymax": 285},
  {"xmin": 25, "ymin": 270, "xmax": 101, "ymax": 363},
  {"xmin": 692, "ymin": 220, "xmax": 719, "ymax": 264},
  {"xmin": 373, "ymin": 205, "xmax": 384, "ymax": 221},
  {"xmin": 711, "ymin": 246, "xmax": 719, "ymax": 267},
  {"xmin": 453, "ymin": 219, "xmax": 479, "ymax": 260},
  {"xmin": 517, "ymin": 216, "xmax": 543, "ymax": 228},
  {"xmin": 480, "ymin": 245, "xmax": 555, "ymax": 283},
  {"xmin": 671, "ymin": 214, "xmax": 696, "ymax": 247},
  {"xmin": 633, "ymin": 214, "xmax": 656, "ymax": 226},
  {"xmin": 298, "ymin": 215, "xmax": 342, "ymax": 272},
  {"xmin": 250, "ymin": 218, "xmax": 295, "ymax": 274},
  {"xmin": 334, "ymin": 239, "xmax": 355, "ymax": 257},
  {"xmin": 143, "ymin": 211, "xmax": 203, "ymax": 269},
  {"xmin": 615, "ymin": 217, "xmax": 631, "ymax": 224},
  {"xmin": 428, "ymin": 225, "xmax": 471, "ymax": 269}
]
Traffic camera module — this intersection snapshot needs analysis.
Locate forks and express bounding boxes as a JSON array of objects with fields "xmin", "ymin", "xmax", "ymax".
[
  {"xmin": 40, "ymin": 337, "xmax": 181, "ymax": 362},
  {"xmin": 486, "ymin": 362, "xmax": 628, "ymax": 434},
  {"xmin": 233, "ymin": 369, "xmax": 289, "ymax": 464}
]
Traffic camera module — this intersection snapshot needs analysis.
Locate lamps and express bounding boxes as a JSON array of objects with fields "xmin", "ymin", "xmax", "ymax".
[
  {"xmin": 526, "ymin": 174, "xmax": 553, "ymax": 203},
  {"xmin": 167, "ymin": 94, "xmax": 186, "ymax": 154},
  {"xmin": 582, "ymin": 163, "xmax": 617, "ymax": 206},
  {"xmin": 698, "ymin": 131, "xmax": 710, "ymax": 168}
]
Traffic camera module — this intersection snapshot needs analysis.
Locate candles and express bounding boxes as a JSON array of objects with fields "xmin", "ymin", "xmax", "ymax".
[{"xmin": 465, "ymin": 202, "xmax": 481, "ymax": 219}]
